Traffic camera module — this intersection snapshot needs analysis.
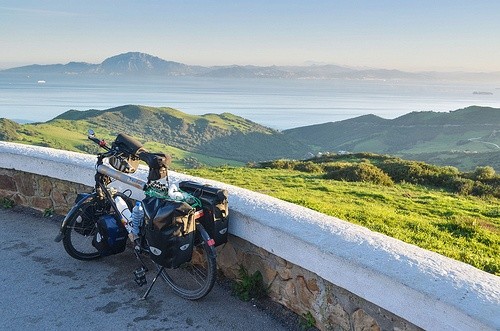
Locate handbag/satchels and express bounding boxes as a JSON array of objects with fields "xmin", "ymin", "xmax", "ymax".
[{"xmin": 110, "ymin": 133, "xmax": 142, "ymax": 173}]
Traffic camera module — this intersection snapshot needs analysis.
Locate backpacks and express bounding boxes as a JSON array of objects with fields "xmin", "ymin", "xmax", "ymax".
[
  {"xmin": 74, "ymin": 193, "xmax": 111, "ymax": 236},
  {"xmin": 179, "ymin": 181, "xmax": 229, "ymax": 248},
  {"xmin": 92, "ymin": 215, "xmax": 127, "ymax": 256},
  {"xmin": 141, "ymin": 194, "xmax": 196, "ymax": 267}
]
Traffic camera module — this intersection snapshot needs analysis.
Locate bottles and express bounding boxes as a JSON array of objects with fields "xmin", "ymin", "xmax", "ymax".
[
  {"xmin": 115, "ymin": 196, "xmax": 131, "ymax": 222},
  {"xmin": 132, "ymin": 202, "xmax": 144, "ymax": 233}
]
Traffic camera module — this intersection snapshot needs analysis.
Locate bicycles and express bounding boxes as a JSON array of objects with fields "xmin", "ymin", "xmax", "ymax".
[{"xmin": 53, "ymin": 128, "xmax": 217, "ymax": 300}]
